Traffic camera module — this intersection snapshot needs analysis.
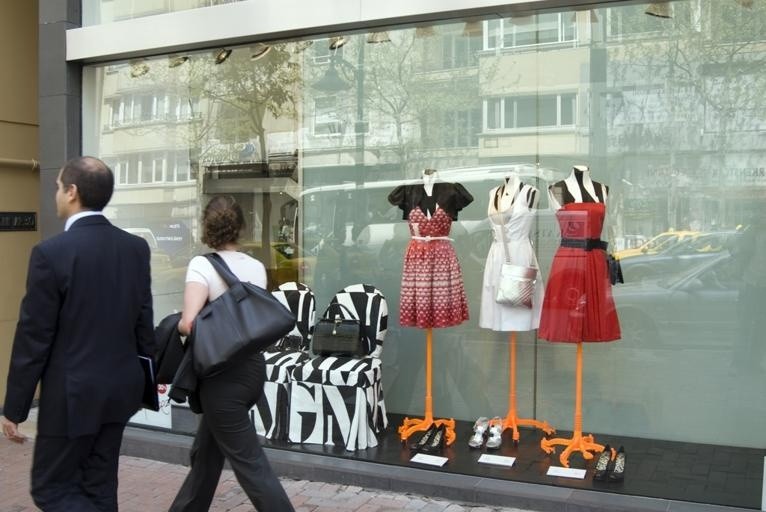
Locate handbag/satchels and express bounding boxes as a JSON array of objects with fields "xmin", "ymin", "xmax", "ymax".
[
  {"xmin": 186, "ymin": 254, "xmax": 296, "ymax": 379},
  {"xmin": 312, "ymin": 302, "xmax": 363, "ymax": 355},
  {"xmin": 496, "ymin": 263, "xmax": 538, "ymax": 309}
]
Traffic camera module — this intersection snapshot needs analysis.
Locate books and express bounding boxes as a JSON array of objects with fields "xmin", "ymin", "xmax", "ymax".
[{"xmin": 136, "ymin": 354, "xmax": 162, "ymax": 414}]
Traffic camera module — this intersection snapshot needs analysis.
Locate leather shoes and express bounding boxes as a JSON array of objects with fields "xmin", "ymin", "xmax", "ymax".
[
  {"xmin": 593, "ymin": 444, "xmax": 627, "ymax": 482},
  {"xmin": 411, "ymin": 423, "xmax": 445, "ymax": 452}
]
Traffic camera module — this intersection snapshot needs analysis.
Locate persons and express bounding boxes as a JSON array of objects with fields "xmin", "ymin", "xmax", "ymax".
[
  {"xmin": 0, "ymin": 152, "xmax": 162, "ymax": 512},
  {"xmin": 727, "ymin": 199, "xmax": 765, "ymax": 377},
  {"xmin": 277, "ymin": 219, "xmax": 290, "ymax": 241},
  {"xmin": 537, "ymin": 163, "xmax": 625, "ymax": 344},
  {"xmin": 477, "ymin": 173, "xmax": 547, "ymax": 336},
  {"xmin": 385, "ymin": 166, "xmax": 478, "ymax": 335},
  {"xmin": 164, "ymin": 192, "xmax": 296, "ymax": 512},
  {"xmin": 312, "ymin": 212, "xmax": 500, "ymax": 419}
]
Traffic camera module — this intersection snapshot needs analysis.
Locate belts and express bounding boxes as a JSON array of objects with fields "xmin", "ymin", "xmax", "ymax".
[{"xmin": 412, "ymin": 235, "xmax": 455, "ymax": 241}]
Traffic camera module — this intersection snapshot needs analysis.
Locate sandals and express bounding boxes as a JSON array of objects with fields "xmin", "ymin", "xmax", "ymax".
[{"xmin": 468, "ymin": 416, "xmax": 504, "ymax": 450}]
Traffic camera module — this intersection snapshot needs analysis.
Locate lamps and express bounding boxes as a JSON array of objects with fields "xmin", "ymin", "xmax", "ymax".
[
  {"xmin": 330, "ymin": 33, "xmax": 348, "ymax": 49},
  {"xmin": 131, "ymin": 58, "xmax": 148, "ymax": 77},
  {"xmin": 645, "ymin": 2, "xmax": 673, "ymax": 18},
  {"xmin": 292, "ymin": 39, "xmax": 312, "ymax": 53},
  {"xmin": 509, "ymin": 14, "xmax": 531, "ymax": 25},
  {"xmin": 250, "ymin": 42, "xmax": 270, "ymax": 61},
  {"xmin": 215, "ymin": 47, "xmax": 230, "ymax": 64},
  {"xmin": 368, "ymin": 29, "xmax": 390, "ymax": 43},
  {"xmin": 571, "ymin": 8, "xmax": 599, "ymax": 23},
  {"xmin": 463, "ymin": 18, "xmax": 483, "ymax": 37},
  {"xmin": 413, "ymin": 25, "xmax": 436, "ymax": 38},
  {"xmin": 167, "ymin": 52, "xmax": 189, "ymax": 67}
]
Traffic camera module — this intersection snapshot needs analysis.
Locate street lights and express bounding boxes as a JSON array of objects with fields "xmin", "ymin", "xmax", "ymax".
[{"xmin": 310, "ymin": 52, "xmax": 375, "ymax": 266}]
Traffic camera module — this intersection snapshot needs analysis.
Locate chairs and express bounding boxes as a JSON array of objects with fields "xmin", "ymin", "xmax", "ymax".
[
  {"xmin": 246, "ymin": 281, "xmax": 315, "ymax": 439},
  {"xmin": 289, "ymin": 284, "xmax": 389, "ymax": 452}
]
